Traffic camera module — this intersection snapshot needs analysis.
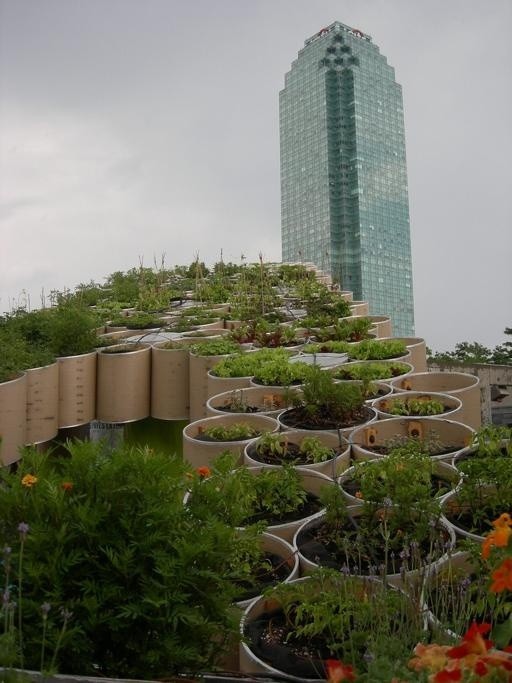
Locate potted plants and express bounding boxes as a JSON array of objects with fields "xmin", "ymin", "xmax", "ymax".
[{"xmin": 0, "ymin": 252, "xmax": 512, "ymax": 683}]
